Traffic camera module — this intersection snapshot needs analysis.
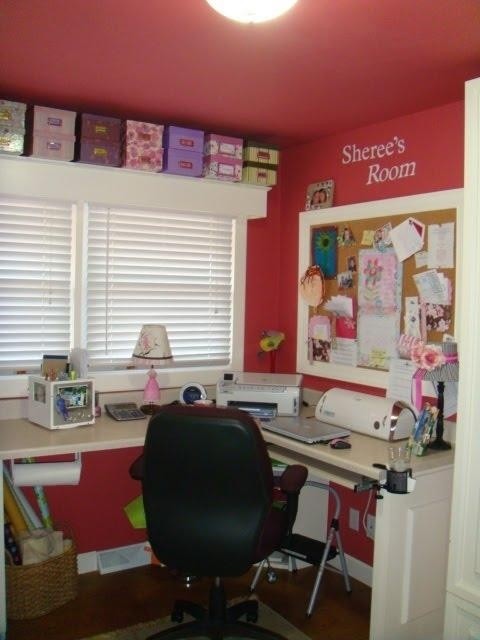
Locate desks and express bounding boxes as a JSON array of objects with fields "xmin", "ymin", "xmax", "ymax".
[{"xmin": 0, "ymin": 397, "xmax": 454, "ymax": 640}]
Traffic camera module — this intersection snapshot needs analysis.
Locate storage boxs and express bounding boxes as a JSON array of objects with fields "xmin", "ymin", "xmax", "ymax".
[
  {"xmin": 122, "ymin": 119, "xmax": 165, "ymax": 172},
  {"xmin": 30, "ymin": 105, "xmax": 77, "ymax": 162},
  {"xmin": 0, "ymin": 99, "xmax": 28, "ymax": 155},
  {"xmin": 162, "ymin": 125, "xmax": 205, "ymax": 176},
  {"xmin": 240, "ymin": 141, "xmax": 280, "ymax": 188},
  {"xmin": 201, "ymin": 133, "xmax": 244, "ymax": 183},
  {"xmin": 79, "ymin": 113, "xmax": 122, "ymax": 166}
]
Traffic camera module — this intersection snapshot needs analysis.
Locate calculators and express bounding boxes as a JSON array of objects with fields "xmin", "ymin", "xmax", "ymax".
[{"xmin": 105, "ymin": 403, "xmax": 146, "ymax": 421}]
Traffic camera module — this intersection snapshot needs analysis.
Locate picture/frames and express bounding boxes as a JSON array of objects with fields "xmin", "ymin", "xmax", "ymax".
[
  {"xmin": 296, "ymin": 189, "xmax": 461, "ymax": 391},
  {"xmin": 404, "ymin": 401, "xmax": 441, "ymax": 457},
  {"xmin": 304, "ymin": 178, "xmax": 334, "ymax": 212}
]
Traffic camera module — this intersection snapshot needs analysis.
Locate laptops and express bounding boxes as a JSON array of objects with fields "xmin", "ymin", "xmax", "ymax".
[{"xmin": 261, "ymin": 417, "xmax": 351, "ymax": 443}]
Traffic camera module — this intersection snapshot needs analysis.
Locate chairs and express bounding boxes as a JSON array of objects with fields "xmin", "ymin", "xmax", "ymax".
[
  {"xmin": 130, "ymin": 405, "xmax": 309, "ymax": 640},
  {"xmin": 252, "ymin": 479, "xmax": 351, "ymax": 615}
]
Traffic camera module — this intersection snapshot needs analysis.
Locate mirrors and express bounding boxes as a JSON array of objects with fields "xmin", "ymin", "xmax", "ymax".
[{"xmin": 178, "ymin": 383, "xmax": 207, "ymax": 405}]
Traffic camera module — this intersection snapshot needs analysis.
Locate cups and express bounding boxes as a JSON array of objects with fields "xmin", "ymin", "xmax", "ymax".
[{"xmin": 388, "ymin": 446, "xmax": 411, "ymax": 472}]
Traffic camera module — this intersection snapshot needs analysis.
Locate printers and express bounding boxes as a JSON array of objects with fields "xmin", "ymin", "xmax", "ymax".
[{"xmin": 216, "ymin": 371, "xmax": 301, "ymax": 419}]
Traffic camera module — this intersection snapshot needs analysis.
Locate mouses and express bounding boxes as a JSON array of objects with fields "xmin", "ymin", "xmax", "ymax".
[{"xmin": 330, "ymin": 439, "xmax": 352, "ymax": 449}]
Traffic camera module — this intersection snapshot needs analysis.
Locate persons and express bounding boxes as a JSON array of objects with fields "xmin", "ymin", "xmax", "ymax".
[
  {"xmin": 56, "ymin": 394, "xmax": 69, "ymax": 422},
  {"xmin": 311, "ymin": 189, "xmax": 326, "ymax": 204}
]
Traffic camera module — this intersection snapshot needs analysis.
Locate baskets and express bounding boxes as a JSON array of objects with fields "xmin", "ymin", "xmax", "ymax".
[{"xmin": 6, "ymin": 520, "xmax": 77, "ymax": 623}]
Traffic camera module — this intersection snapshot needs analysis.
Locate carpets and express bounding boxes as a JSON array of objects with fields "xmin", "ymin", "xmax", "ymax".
[{"xmin": 84, "ymin": 595, "xmax": 314, "ymax": 640}]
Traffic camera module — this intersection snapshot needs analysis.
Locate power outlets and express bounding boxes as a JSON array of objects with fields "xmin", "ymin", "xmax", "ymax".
[
  {"xmin": 349, "ymin": 510, "xmax": 358, "ymax": 530},
  {"xmin": 366, "ymin": 515, "xmax": 376, "ymax": 541}
]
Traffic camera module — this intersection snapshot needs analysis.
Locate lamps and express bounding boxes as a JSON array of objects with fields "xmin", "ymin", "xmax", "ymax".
[
  {"xmin": 414, "ymin": 342, "xmax": 458, "ymax": 455},
  {"xmin": 132, "ymin": 325, "xmax": 173, "ymax": 416}
]
